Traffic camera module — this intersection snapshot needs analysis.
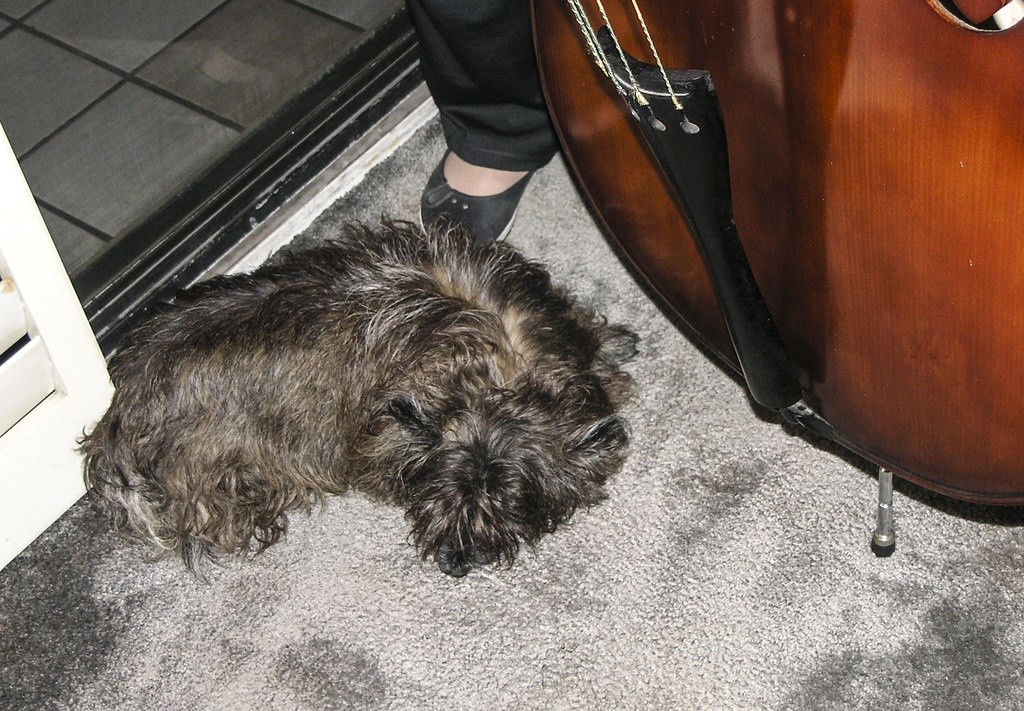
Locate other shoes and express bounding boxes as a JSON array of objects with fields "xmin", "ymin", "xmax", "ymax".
[{"xmin": 418, "ymin": 146, "xmax": 537, "ymax": 247}]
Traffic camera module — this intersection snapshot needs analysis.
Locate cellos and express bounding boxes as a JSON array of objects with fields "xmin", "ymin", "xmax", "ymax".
[{"xmin": 530, "ymin": 0, "xmax": 1024, "ymax": 553}]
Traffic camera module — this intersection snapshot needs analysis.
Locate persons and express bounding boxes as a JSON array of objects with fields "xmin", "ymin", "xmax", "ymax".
[{"xmin": 404, "ymin": 0, "xmax": 554, "ymax": 242}]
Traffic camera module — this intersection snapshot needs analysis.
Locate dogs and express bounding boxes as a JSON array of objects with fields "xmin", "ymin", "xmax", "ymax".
[{"xmin": 75, "ymin": 213, "xmax": 639, "ymax": 578}]
